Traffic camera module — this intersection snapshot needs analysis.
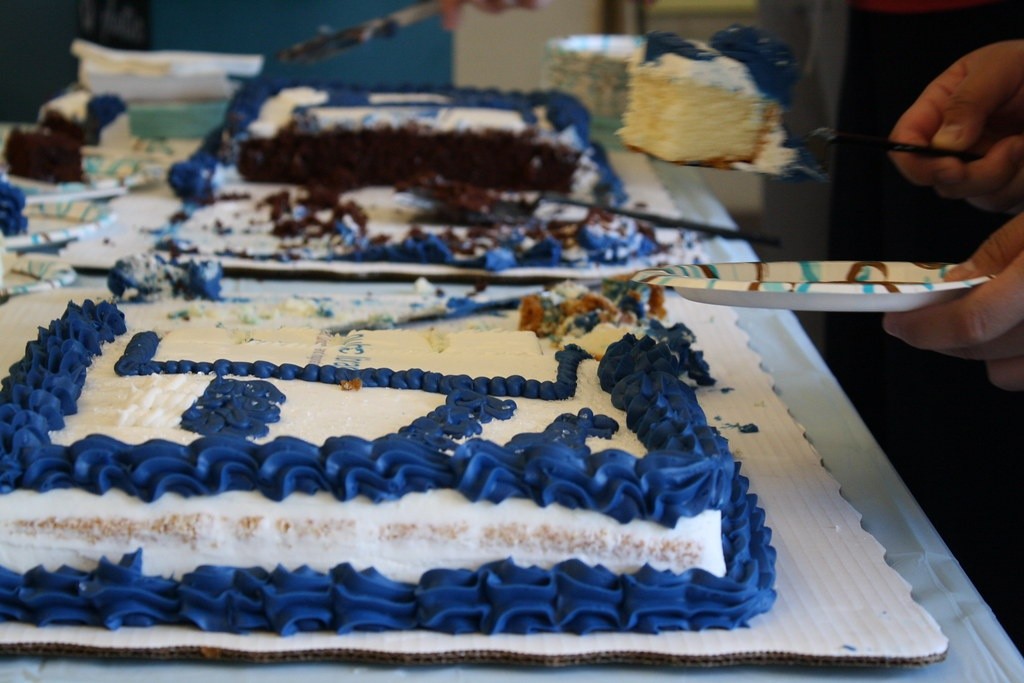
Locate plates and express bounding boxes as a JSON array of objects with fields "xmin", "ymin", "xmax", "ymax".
[{"xmin": 631, "ymin": 261, "xmax": 996, "ymax": 311}]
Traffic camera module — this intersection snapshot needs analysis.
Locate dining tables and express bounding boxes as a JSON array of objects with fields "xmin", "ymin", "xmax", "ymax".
[{"xmin": 0, "ymin": 116, "xmax": 1024, "ymax": 683}]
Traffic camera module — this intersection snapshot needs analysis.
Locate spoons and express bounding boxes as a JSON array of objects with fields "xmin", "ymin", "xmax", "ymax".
[{"xmin": 783, "ymin": 126, "xmax": 984, "ymax": 161}]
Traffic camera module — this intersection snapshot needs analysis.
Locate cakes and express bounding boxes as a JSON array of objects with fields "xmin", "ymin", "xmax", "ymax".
[
  {"xmin": 619, "ymin": 43, "xmax": 772, "ymax": 167},
  {"xmin": 0, "ymin": 76, "xmax": 777, "ymax": 636}
]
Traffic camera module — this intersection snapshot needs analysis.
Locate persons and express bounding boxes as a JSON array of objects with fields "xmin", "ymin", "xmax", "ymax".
[{"xmin": 885, "ymin": 40, "xmax": 1024, "ymax": 391}]
